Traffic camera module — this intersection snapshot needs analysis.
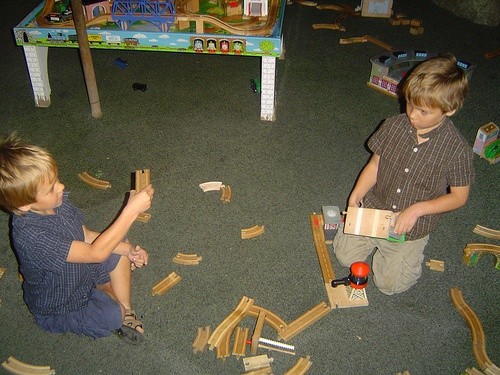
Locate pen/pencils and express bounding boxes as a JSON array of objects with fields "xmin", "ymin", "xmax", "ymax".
[{"xmin": 246, "ymin": 339, "xmax": 295, "ymax": 356}]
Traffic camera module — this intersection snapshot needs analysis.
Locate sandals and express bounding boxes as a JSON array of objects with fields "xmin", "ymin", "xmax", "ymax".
[{"xmin": 112, "ymin": 309, "xmax": 144, "ymax": 346}]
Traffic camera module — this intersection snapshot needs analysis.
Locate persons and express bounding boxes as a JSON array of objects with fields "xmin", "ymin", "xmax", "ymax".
[
  {"xmin": 0, "ymin": 130, "xmax": 154, "ymax": 345},
  {"xmin": 331, "ymin": 55, "xmax": 474, "ymax": 296}
]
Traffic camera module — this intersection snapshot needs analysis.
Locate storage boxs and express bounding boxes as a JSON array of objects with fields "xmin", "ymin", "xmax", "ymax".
[{"xmin": 361, "ymin": 0, "xmax": 393, "ymax": 18}]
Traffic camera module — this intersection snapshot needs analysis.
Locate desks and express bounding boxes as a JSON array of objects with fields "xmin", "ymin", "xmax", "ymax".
[{"xmin": 14, "ymin": 0, "xmax": 287, "ymax": 122}]
